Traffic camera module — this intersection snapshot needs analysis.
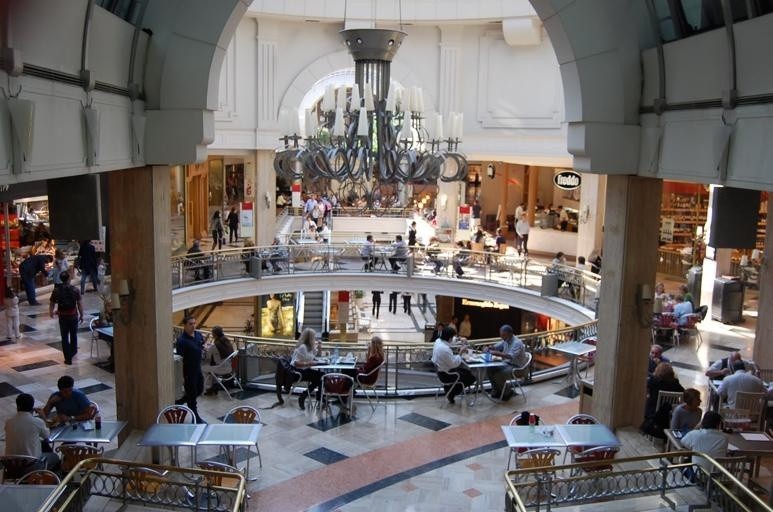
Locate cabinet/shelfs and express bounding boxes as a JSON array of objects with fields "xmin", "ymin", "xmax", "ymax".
[
  {"xmin": 660, "ymin": 193, "xmax": 711, "ymax": 250},
  {"xmin": 710, "ymin": 275, "xmax": 746, "ymax": 325}
]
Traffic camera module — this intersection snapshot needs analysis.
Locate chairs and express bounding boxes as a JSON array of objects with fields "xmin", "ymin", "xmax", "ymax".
[
  {"xmin": 705, "ymin": 456, "xmax": 747, "ymax": 512},
  {"xmin": 55, "ymin": 444, "xmax": 104, "ymax": 479},
  {"xmin": 156, "ymin": 405, "xmax": 197, "ymax": 476},
  {"xmin": 720, "ymin": 408, "xmax": 754, "ymax": 478},
  {"xmin": 202, "ymin": 350, "xmax": 244, "ymax": 400},
  {"xmin": 736, "ymin": 391, "xmax": 768, "ymax": 477},
  {"xmin": 355, "ymin": 360, "xmax": 386, "ymax": 411},
  {"xmin": 210, "ymin": 254, "xmax": 225, "ymax": 278},
  {"xmin": 497, "ymin": 352, "xmax": 532, "ymax": 402},
  {"xmin": 320, "ymin": 373, "xmax": 355, "ymax": 419},
  {"xmin": 0, "ymin": 454, "xmax": 49, "ymax": 485},
  {"xmin": 507, "ymin": 414, "xmax": 555, "ymax": 469},
  {"xmin": 219, "ymin": 406, "xmax": 263, "ymax": 481},
  {"xmin": 16, "ymin": 470, "xmax": 61, "ymax": 486},
  {"xmin": 358, "ymin": 248, "xmax": 375, "ymax": 272},
  {"xmin": 195, "ymin": 461, "xmax": 250, "ymax": 507},
  {"xmin": 176, "ymin": 257, "xmax": 196, "ymax": 287},
  {"xmin": 576, "ymin": 337, "xmax": 597, "ymax": 377},
  {"xmin": 695, "ymin": 306, "xmax": 708, "ymax": 343},
  {"xmin": 647, "ymin": 390, "xmax": 683, "ymax": 446},
  {"xmin": 514, "ymin": 448, "xmax": 561, "ymax": 500},
  {"xmin": 280, "ymin": 355, "xmax": 317, "ymax": 409},
  {"xmin": 119, "ymin": 465, "xmax": 170, "ymax": 502},
  {"xmin": 675, "ymin": 312, "xmax": 700, "ymax": 350},
  {"xmin": 239, "ymin": 254, "xmax": 253, "ymax": 275},
  {"xmin": 562, "ymin": 414, "xmax": 601, "ymax": 470},
  {"xmin": 71, "ymin": 401, "xmax": 99, "ymax": 452},
  {"xmin": 653, "ymin": 311, "xmax": 677, "ymax": 348},
  {"xmin": 330, "ymin": 248, "xmax": 345, "ymax": 271},
  {"xmin": 425, "ymin": 358, "xmax": 471, "ymax": 409},
  {"xmin": 89, "ymin": 316, "xmax": 102, "ymax": 360},
  {"xmin": 568, "ymin": 446, "xmax": 620, "ymax": 492},
  {"xmin": 418, "ymin": 250, "xmax": 436, "ymax": 275}
]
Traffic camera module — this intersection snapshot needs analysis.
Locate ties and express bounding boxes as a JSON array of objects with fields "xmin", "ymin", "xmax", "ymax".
[
  {"xmin": 439, "ymin": 330, "xmax": 441, "ymax": 336},
  {"xmin": 309, "ymin": 221, "xmax": 311, "ymax": 229}
]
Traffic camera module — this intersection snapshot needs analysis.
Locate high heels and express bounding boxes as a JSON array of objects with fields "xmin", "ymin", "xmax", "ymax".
[
  {"xmin": 298, "ymin": 391, "xmax": 307, "ymax": 410},
  {"xmin": 206, "ymin": 383, "xmax": 218, "ymax": 391},
  {"xmin": 331, "ymin": 396, "xmax": 347, "ymax": 409},
  {"xmin": 204, "ymin": 387, "xmax": 219, "ymax": 395},
  {"xmin": 353, "ymin": 390, "xmax": 357, "ymax": 397},
  {"xmin": 316, "ymin": 391, "xmax": 329, "ymax": 408}
]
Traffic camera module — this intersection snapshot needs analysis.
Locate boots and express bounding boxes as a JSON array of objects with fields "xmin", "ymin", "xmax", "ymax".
[
  {"xmin": 235, "ymin": 235, "xmax": 238, "ymax": 241},
  {"xmin": 212, "ymin": 242, "xmax": 216, "ymax": 250},
  {"xmin": 191, "ymin": 409, "xmax": 207, "ymax": 425},
  {"xmin": 175, "ymin": 399, "xmax": 179, "ymax": 405},
  {"xmin": 219, "ymin": 239, "xmax": 221, "ymax": 249},
  {"xmin": 230, "ymin": 235, "xmax": 232, "ymax": 243}
]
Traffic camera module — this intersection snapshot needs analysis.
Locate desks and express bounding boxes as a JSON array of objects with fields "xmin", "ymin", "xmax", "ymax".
[
  {"xmin": 547, "ymin": 340, "xmax": 596, "ymax": 391},
  {"xmin": 198, "ymin": 423, "xmax": 262, "ymax": 465},
  {"xmin": 459, "ymin": 353, "xmax": 508, "ymax": 406},
  {"xmin": 311, "ymin": 356, "xmax": 359, "ymax": 373},
  {"xmin": 296, "ymin": 239, "xmax": 319, "ymax": 260},
  {"xmin": 706, "ymin": 378, "xmax": 771, "ymax": 415},
  {"xmin": 96, "ymin": 326, "xmax": 113, "ymax": 363},
  {"xmin": 1, "ymin": 420, "xmax": 66, "ymax": 444},
  {"xmin": 663, "ymin": 428, "xmax": 773, "ymax": 456},
  {"xmin": 370, "ymin": 250, "xmax": 392, "ymax": 271},
  {"xmin": 501, "ymin": 425, "xmax": 566, "ymax": 452},
  {"xmin": 136, "ymin": 424, "xmax": 207, "ymax": 466},
  {"xmin": 54, "ymin": 419, "xmax": 129, "ymax": 446},
  {"xmin": 556, "ymin": 423, "xmax": 623, "ymax": 450}
]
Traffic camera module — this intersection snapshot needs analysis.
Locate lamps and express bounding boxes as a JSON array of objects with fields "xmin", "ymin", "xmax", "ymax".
[{"xmin": 273, "ymin": 2, "xmax": 471, "ymax": 217}]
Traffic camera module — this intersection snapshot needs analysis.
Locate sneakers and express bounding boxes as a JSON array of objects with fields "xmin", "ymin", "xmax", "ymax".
[
  {"xmin": 5, "ymin": 336, "xmax": 12, "ymax": 339},
  {"xmin": 501, "ymin": 389, "xmax": 513, "ymax": 400},
  {"xmin": 16, "ymin": 334, "xmax": 22, "ymax": 339},
  {"xmin": 491, "ymin": 388, "xmax": 501, "ymax": 397},
  {"xmin": 196, "ymin": 277, "xmax": 202, "ymax": 280},
  {"xmin": 206, "ymin": 275, "xmax": 212, "ymax": 278}
]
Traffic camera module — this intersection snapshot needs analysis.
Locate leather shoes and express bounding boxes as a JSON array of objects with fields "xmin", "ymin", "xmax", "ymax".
[
  {"xmin": 72, "ymin": 351, "xmax": 77, "ymax": 355},
  {"xmin": 365, "ymin": 264, "xmax": 367, "ymax": 272},
  {"xmin": 444, "ymin": 385, "xmax": 456, "ymax": 404},
  {"xmin": 94, "ymin": 288, "xmax": 97, "ymax": 290},
  {"xmin": 82, "ymin": 293, "xmax": 83, "ymax": 294},
  {"xmin": 64, "ymin": 360, "xmax": 71, "ymax": 364},
  {"xmin": 275, "ymin": 268, "xmax": 281, "ymax": 271},
  {"xmin": 262, "ymin": 267, "xmax": 267, "ymax": 270},
  {"xmin": 368, "ymin": 265, "xmax": 372, "ymax": 272},
  {"xmin": 30, "ymin": 303, "xmax": 41, "ymax": 305}
]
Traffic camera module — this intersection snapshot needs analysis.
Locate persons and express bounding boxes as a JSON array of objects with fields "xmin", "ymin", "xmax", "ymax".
[
  {"xmin": 183, "ymin": 239, "xmax": 210, "ymax": 280},
  {"xmin": 264, "ymin": 294, "xmax": 286, "ymax": 335},
  {"xmin": 430, "ymin": 327, "xmax": 473, "ymax": 404},
  {"xmin": 401, "ymin": 292, "xmax": 412, "ymax": 315},
  {"xmin": 460, "ymin": 314, "xmax": 473, "ymax": 338},
  {"xmin": 357, "ymin": 196, "xmax": 366, "ymax": 209},
  {"xmin": 489, "ymin": 227, "xmax": 507, "ymax": 256},
  {"xmin": 0, "ymin": 275, "xmax": 8, "ymax": 344},
  {"xmin": 457, "ymin": 214, "xmax": 470, "ymax": 231},
  {"xmin": 678, "ymin": 284, "xmax": 695, "ymax": 305},
  {"xmin": 648, "ymin": 344, "xmax": 673, "ymax": 374},
  {"xmin": 305, "ymin": 194, "xmax": 317, "ymax": 213},
  {"xmin": 302, "ymin": 190, "xmax": 309, "ymax": 201},
  {"xmin": 454, "ymin": 240, "xmax": 470, "ymax": 277},
  {"xmin": 405, "ymin": 221, "xmax": 417, "ymax": 256},
  {"xmin": 551, "ymin": 251, "xmax": 567, "ymax": 266},
  {"xmin": 205, "ymin": 325, "xmax": 233, "ymax": 396},
  {"xmin": 308, "ymin": 225, "xmax": 317, "ymax": 239},
  {"xmin": 514, "ymin": 202, "xmax": 526, "ymax": 246},
  {"xmin": 446, "ymin": 316, "xmax": 458, "ymax": 334},
  {"xmin": 268, "ymin": 236, "xmax": 288, "ymax": 274},
  {"xmin": 50, "ymin": 248, "xmax": 71, "ymax": 296},
  {"xmin": 705, "ymin": 351, "xmax": 743, "ymax": 413},
  {"xmin": 554, "ymin": 204, "xmax": 568, "ymax": 226},
  {"xmin": 471, "ymin": 199, "xmax": 481, "ymax": 220},
  {"xmin": 290, "ymin": 328, "xmax": 322, "ymax": 411},
  {"xmin": 41, "ymin": 375, "xmax": 91, "ymax": 427},
  {"xmin": 304, "ymin": 216, "xmax": 317, "ymax": 231},
  {"xmin": 515, "ymin": 212, "xmax": 529, "ymax": 255},
  {"xmin": 425, "ymin": 237, "xmax": 443, "ymax": 272},
  {"xmin": 4, "ymin": 393, "xmax": 60, "ymax": 473},
  {"xmin": 670, "ymin": 388, "xmax": 702, "ymax": 433},
  {"xmin": 208, "ymin": 210, "xmax": 224, "ymax": 255},
  {"xmin": 19, "ymin": 254, "xmax": 53, "ymax": 304},
  {"xmin": 545, "ymin": 203, "xmax": 555, "ymax": 216},
  {"xmin": 331, "ymin": 335, "xmax": 384, "ymax": 420},
  {"xmin": 655, "ymin": 282, "xmax": 672, "ymax": 303},
  {"xmin": 277, "ymin": 192, "xmax": 291, "ymax": 206},
  {"xmin": 673, "ymin": 294, "xmax": 692, "ymax": 333},
  {"xmin": 176, "ymin": 316, "xmax": 208, "ymax": 426},
  {"xmin": 494, "ymin": 200, "xmax": 502, "ymax": 229},
  {"xmin": 646, "ymin": 362, "xmax": 685, "ymax": 421},
  {"xmin": 664, "ymin": 293, "xmax": 675, "ymax": 313},
  {"xmin": 387, "ymin": 290, "xmax": 399, "ymax": 314},
  {"xmin": 429, "ymin": 211, "xmax": 437, "ymax": 224},
  {"xmin": 320, "ymin": 221, "xmax": 329, "ymax": 243},
  {"xmin": 315, "ymin": 196, "xmax": 323, "ymax": 203},
  {"xmin": 716, "ymin": 360, "xmax": 769, "ymax": 424},
  {"xmin": 587, "ymin": 254, "xmax": 602, "ymax": 275},
  {"xmin": 428, "ymin": 323, "xmax": 449, "ymax": 342},
  {"xmin": 388, "ymin": 235, "xmax": 407, "ymax": 271},
  {"xmin": 482, "ymin": 325, "xmax": 525, "ymax": 399},
  {"xmin": 325, "ymin": 189, "xmax": 340, "ymax": 207},
  {"xmin": 680, "ymin": 411, "xmax": 728, "ymax": 479},
  {"xmin": 5, "ymin": 287, "xmax": 22, "ymax": 342},
  {"xmin": 224, "ymin": 206, "xmax": 240, "ymax": 243},
  {"xmin": 471, "ymin": 229, "xmax": 486, "ymax": 251},
  {"xmin": 361, "ymin": 234, "xmax": 379, "ymax": 272},
  {"xmin": 568, "ymin": 256, "xmax": 589, "ymax": 298},
  {"xmin": 49, "ymin": 270, "xmax": 84, "ymax": 365},
  {"xmin": 368, "ymin": 290, "xmax": 385, "ymax": 319}
]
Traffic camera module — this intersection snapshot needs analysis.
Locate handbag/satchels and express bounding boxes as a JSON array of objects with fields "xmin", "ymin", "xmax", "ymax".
[
  {"xmin": 644, "ymin": 402, "xmax": 672, "ymax": 440},
  {"xmin": 317, "ymin": 218, "xmax": 322, "ymax": 227},
  {"xmin": 217, "ymin": 217, "xmax": 222, "ymax": 237}
]
